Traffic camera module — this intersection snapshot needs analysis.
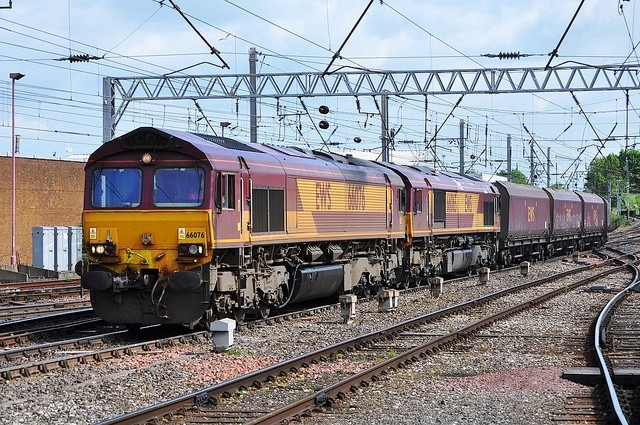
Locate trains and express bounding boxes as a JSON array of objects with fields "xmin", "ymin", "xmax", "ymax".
[{"xmin": 74, "ymin": 125, "xmax": 609, "ymax": 335}]
